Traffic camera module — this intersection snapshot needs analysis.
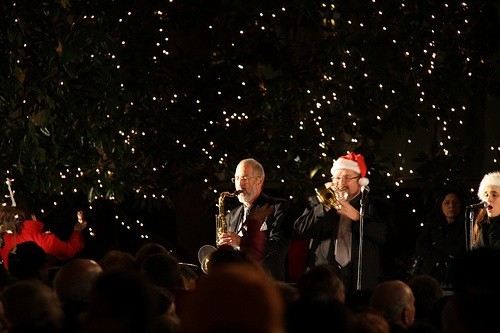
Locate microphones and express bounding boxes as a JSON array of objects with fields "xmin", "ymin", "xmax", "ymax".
[
  {"xmin": 467, "ymin": 202, "xmax": 487, "ymax": 209},
  {"xmin": 361, "ymin": 186, "xmax": 370, "ymax": 205}
]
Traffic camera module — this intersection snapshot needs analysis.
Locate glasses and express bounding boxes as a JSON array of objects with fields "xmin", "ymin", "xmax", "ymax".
[
  {"xmin": 232, "ymin": 176, "xmax": 262, "ymax": 184},
  {"xmin": 330, "ymin": 176, "xmax": 360, "ymax": 183}
]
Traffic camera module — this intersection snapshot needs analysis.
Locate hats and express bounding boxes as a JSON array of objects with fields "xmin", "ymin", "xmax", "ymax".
[
  {"xmin": 330, "ymin": 153, "xmax": 369, "ymax": 185},
  {"xmin": 477, "ymin": 173, "xmax": 500, "ymax": 200}
]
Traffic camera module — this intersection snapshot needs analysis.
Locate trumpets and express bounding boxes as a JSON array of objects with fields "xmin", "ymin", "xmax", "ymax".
[{"xmin": 317, "ymin": 181, "xmax": 350, "ymax": 211}]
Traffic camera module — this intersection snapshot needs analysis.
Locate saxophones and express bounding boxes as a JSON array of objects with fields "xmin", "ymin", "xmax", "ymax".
[
  {"xmin": 199, "ymin": 188, "xmax": 244, "ymax": 273},
  {"xmin": 468, "ymin": 207, "xmax": 489, "ymax": 247}
]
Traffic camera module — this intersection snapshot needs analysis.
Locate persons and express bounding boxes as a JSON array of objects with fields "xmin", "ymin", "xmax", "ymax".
[
  {"xmin": 424, "ymin": 189, "xmax": 466, "ymax": 234},
  {"xmin": 0, "ymin": 201, "xmax": 87, "ymax": 271},
  {"xmin": 217, "ymin": 159, "xmax": 295, "ymax": 281},
  {"xmin": 295, "ymin": 153, "xmax": 387, "ymax": 306},
  {"xmin": 0, "ymin": 244, "xmax": 500, "ymax": 333},
  {"xmin": 470, "ymin": 171, "xmax": 500, "ymax": 266}
]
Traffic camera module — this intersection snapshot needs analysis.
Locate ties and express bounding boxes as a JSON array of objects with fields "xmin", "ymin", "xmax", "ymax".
[{"xmin": 335, "ymin": 213, "xmax": 352, "ymax": 267}]
